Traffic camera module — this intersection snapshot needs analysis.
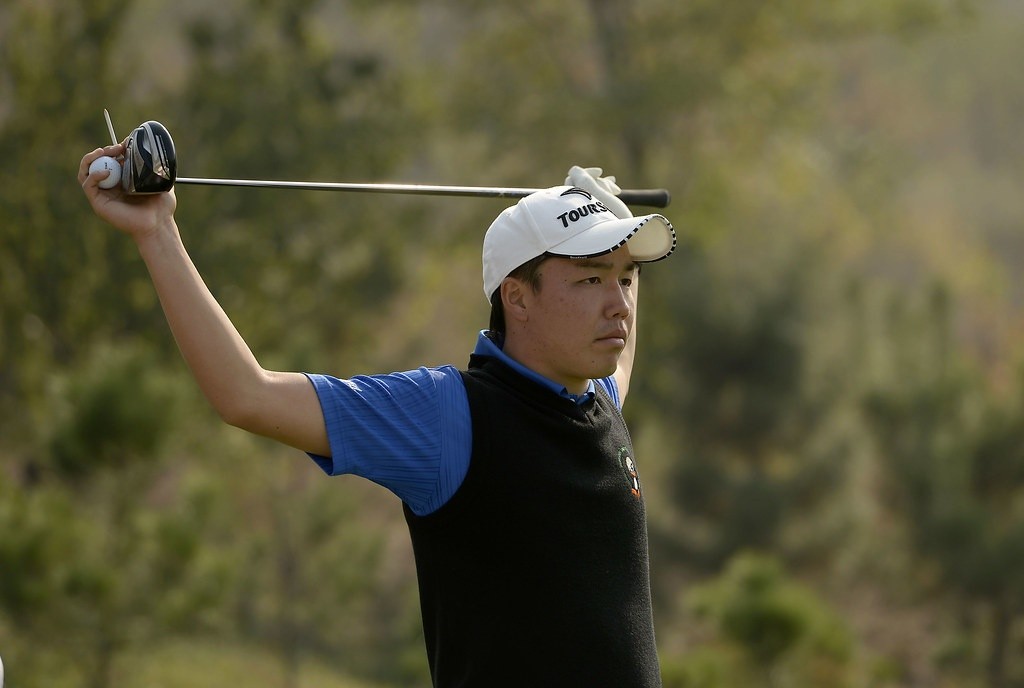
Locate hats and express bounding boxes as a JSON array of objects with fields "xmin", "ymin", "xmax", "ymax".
[{"xmin": 482, "ymin": 186, "xmax": 678, "ymax": 306}]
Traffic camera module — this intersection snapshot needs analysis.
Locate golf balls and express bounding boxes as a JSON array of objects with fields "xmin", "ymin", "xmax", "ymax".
[{"xmin": 88, "ymin": 155, "xmax": 123, "ymax": 189}]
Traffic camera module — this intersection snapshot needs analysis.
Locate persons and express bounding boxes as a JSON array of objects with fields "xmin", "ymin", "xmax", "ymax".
[{"xmin": 77, "ymin": 142, "xmax": 678, "ymax": 688}]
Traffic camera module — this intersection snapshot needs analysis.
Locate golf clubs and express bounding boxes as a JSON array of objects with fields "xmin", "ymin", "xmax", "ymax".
[{"xmin": 120, "ymin": 121, "xmax": 670, "ymax": 210}]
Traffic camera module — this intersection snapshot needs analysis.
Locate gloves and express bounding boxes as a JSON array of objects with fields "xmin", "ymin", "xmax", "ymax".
[{"xmin": 562, "ymin": 166, "xmax": 633, "ymax": 218}]
